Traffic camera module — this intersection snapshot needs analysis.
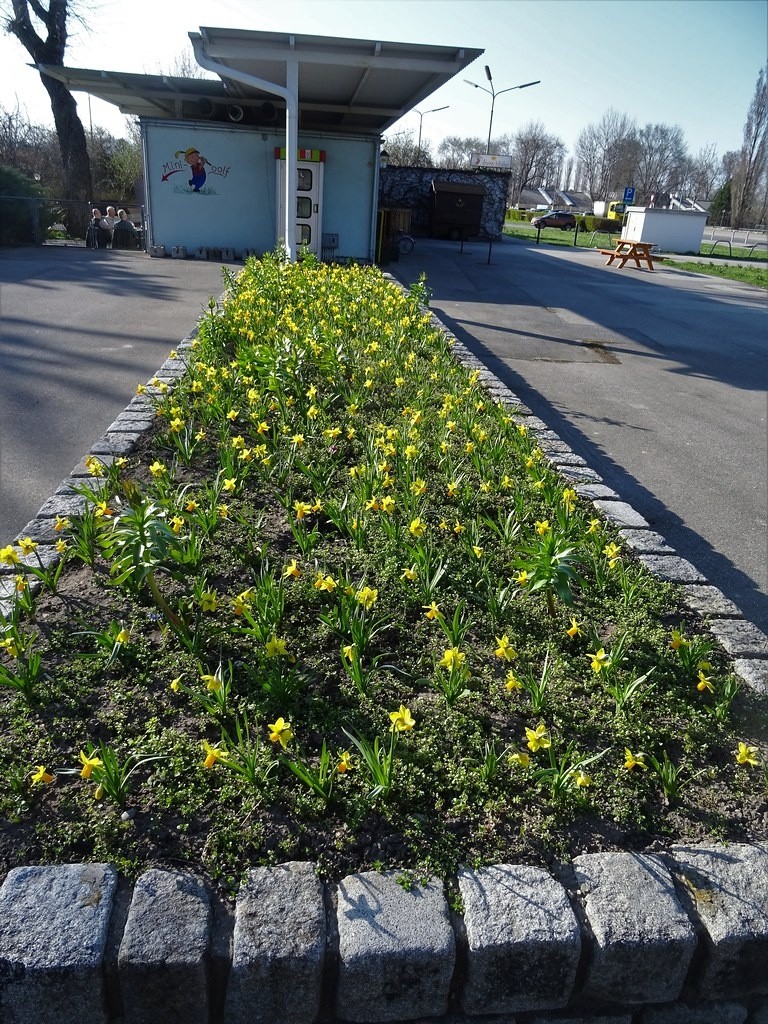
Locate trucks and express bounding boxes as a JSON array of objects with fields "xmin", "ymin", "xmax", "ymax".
[{"xmin": 593, "ymin": 199, "xmax": 627, "ymax": 226}]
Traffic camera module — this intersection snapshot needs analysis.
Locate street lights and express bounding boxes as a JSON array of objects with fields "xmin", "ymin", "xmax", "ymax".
[
  {"xmin": 406, "ymin": 105, "xmax": 450, "ymax": 167},
  {"xmin": 463, "ymin": 65, "xmax": 542, "ymax": 156},
  {"xmin": 671, "ymin": 153, "xmax": 688, "ymax": 211}
]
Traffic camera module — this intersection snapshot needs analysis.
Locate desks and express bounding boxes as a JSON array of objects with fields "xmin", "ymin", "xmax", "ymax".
[{"xmin": 599, "ymin": 238, "xmax": 664, "ymax": 271}]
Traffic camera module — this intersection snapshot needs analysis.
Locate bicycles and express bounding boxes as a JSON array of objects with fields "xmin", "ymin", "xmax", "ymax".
[{"xmin": 397, "ymin": 230, "xmax": 416, "ymax": 255}]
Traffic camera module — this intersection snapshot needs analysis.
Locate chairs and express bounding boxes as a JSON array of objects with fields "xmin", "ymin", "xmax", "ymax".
[
  {"xmin": 85, "ymin": 226, "xmax": 112, "ymax": 248},
  {"xmin": 112, "ymin": 228, "xmax": 137, "ymax": 249}
]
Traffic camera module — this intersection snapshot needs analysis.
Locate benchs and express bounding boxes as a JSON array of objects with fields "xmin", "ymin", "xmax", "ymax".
[
  {"xmin": 638, "ymin": 252, "xmax": 670, "ymax": 259},
  {"xmin": 596, "ymin": 248, "xmax": 628, "ymax": 257}
]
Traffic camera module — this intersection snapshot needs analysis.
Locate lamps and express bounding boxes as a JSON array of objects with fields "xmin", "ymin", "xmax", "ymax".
[{"xmin": 380, "ymin": 149, "xmax": 390, "ymax": 170}]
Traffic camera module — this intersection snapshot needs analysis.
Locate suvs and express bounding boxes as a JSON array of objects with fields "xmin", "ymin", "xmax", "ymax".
[{"xmin": 531, "ymin": 211, "xmax": 576, "ymax": 231}]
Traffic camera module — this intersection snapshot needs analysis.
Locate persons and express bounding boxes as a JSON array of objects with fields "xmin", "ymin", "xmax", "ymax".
[
  {"xmin": 85, "ymin": 206, "xmax": 118, "ymax": 249},
  {"xmin": 112, "ymin": 209, "xmax": 138, "ymax": 249}
]
